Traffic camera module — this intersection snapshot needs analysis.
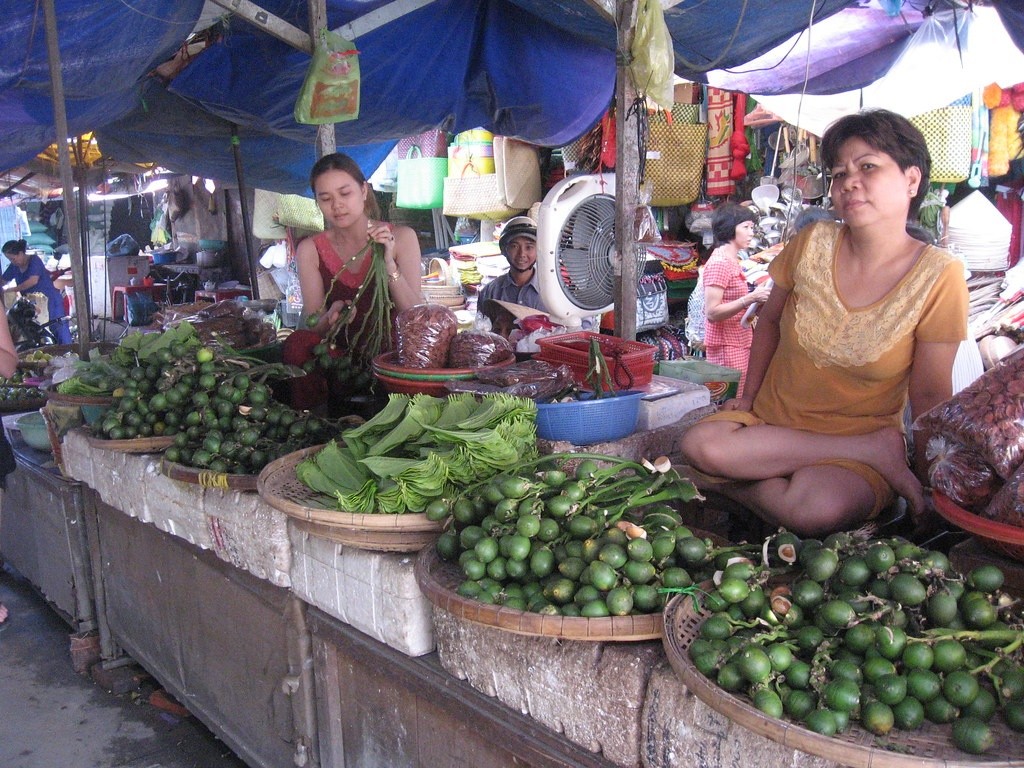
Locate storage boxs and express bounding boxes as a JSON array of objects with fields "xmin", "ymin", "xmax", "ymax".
[
  {"xmin": 536, "ymin": 172, "xmax": 647, "ymax": 327},
  {"xmin": 442, "ymin": 128, "xmax": 526, "ymax": 221},
  {"xmin": 602, "ymin": 359, "xmax": 742, "ymax": 434},
  {"xmin": 270, "ymin": 266, "xmax": 305, "ymax": 329},
  {"xmin": 59, "ymin": 429, "xmax": 429, "ymax": 663},
  {"xmin": 644, "ymin": 78, "xmax": 703, "ymax": 126}
]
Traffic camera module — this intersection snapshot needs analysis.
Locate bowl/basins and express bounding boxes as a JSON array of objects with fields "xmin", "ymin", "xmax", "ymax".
[
  {"xmin": 13, "ymin": 412, "xmax": 53, "ymax": 452},
  {"xmin": 371, "ymin": 349, "xmax": 516, "ymax": 398}
]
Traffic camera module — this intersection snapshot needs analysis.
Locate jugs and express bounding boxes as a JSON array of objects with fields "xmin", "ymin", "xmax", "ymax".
[{"xmin": 203, "ymin": 279, "xmax": 216, "ymax": 292}]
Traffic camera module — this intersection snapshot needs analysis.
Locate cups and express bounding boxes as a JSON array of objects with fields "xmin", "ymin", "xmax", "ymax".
[{"xmin": 130, "ymin": 277, "xmax": 137, "ymax": 286}]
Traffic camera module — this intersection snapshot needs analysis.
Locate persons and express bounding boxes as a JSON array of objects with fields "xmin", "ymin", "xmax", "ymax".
[
  {"xmin": 297, "ymin": 152, "xmax": 423, "ymax": 362},
  {"xmin": 704, "ymin": 203, "xmax": 936, "ymax": 397},
  {"xmin": 0, "ymin": 296, "xmax": 18, "ymax": 628},
  {"xmin": 0, "ymin": 239, "xmax": 73, "ymax": 344},
  {"xmin": 477, "ymin": 216, "xmax": 551, "ymax": 341},
  {"xmin": 680, "ymin": 109, "xmax": 970, "ymax": 539}
]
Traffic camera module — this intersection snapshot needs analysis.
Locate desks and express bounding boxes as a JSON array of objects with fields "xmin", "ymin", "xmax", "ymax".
[
  {"xmin": 112, "ymin": 283, "xmax": 174, "ymax": 323},
  {"xmin": 148, "ymin": 261, "xmax": 239, "ymax": 292},
  {"xmin": 194, "ymin": 290, "xmax": 251, "ymax": 303}
]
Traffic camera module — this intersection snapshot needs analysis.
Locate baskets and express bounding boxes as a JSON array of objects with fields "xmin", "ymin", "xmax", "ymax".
[
  {"xmin": 371, "ymin": 350, "xmax": 516, "ymax": 399},
  {"xmin": 395, "ymin": 147, "xmax": 449, "ymax": 211},
  {"xmin": 422, "ymin": 258, "xmax": 464, "ymax": 307},
  {"xmin": 534, "ymin": 389, "xmax": 646, "ymax": 444},
  {"xmin": 531, "ymin": 331, "xmax": 659, "ymax": 393},
  {"xmin": 443, "ymin": 163, "xmax": 522, "ymax": 222}
]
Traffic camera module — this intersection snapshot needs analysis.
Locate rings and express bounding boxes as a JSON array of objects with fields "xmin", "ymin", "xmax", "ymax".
[{"xmin": 388, "ymin": 235, "xmax": 394, "ymax": 241}]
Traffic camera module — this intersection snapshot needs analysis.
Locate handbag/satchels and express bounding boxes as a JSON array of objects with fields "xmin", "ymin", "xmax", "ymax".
[
  {"xmin": 639, "ymin": 102, "xmax": 708, "ymax": 207},
  {"xmin": 635, "ymin": 276, "xmax": 668, "ymax": 332},
  {"xmin": 907, "ymin": 97, "xmax": 977, "ymax": 183},
  {"xmin": 285, "ymin": 228, "xmax": 306, "ymax": 314},
  {"xmin": 278, "ymin": 192, "xmax": 325, "ymax": 233},
  {"xmin": 685, "ymin": 264, "xmax": 708, "ymax": 344}
]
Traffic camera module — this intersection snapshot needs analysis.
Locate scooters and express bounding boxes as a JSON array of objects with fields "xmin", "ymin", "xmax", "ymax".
[{"xmin": 5, "ymin": 297, "xmax": 73, "ymax": 354}]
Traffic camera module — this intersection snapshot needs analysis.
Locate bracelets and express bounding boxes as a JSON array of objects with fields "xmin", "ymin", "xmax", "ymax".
[
  {"xmin": 387, "ymin": 262, "xmax": 402, "ymax": 282},
  {"xmin": 739, "ymin": 298, "xmax": 748, "ymax": 309}
]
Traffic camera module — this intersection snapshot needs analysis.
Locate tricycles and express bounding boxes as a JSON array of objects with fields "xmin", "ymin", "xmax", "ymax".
[{"xmin": 148, "ymin": 269, "xmax": 205, "ymax": 334}]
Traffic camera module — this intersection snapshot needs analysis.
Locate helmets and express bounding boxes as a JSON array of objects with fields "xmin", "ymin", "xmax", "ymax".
[{"xmin": 499, "ymin": 216, "xmax": 537, "ymax": 253}]
